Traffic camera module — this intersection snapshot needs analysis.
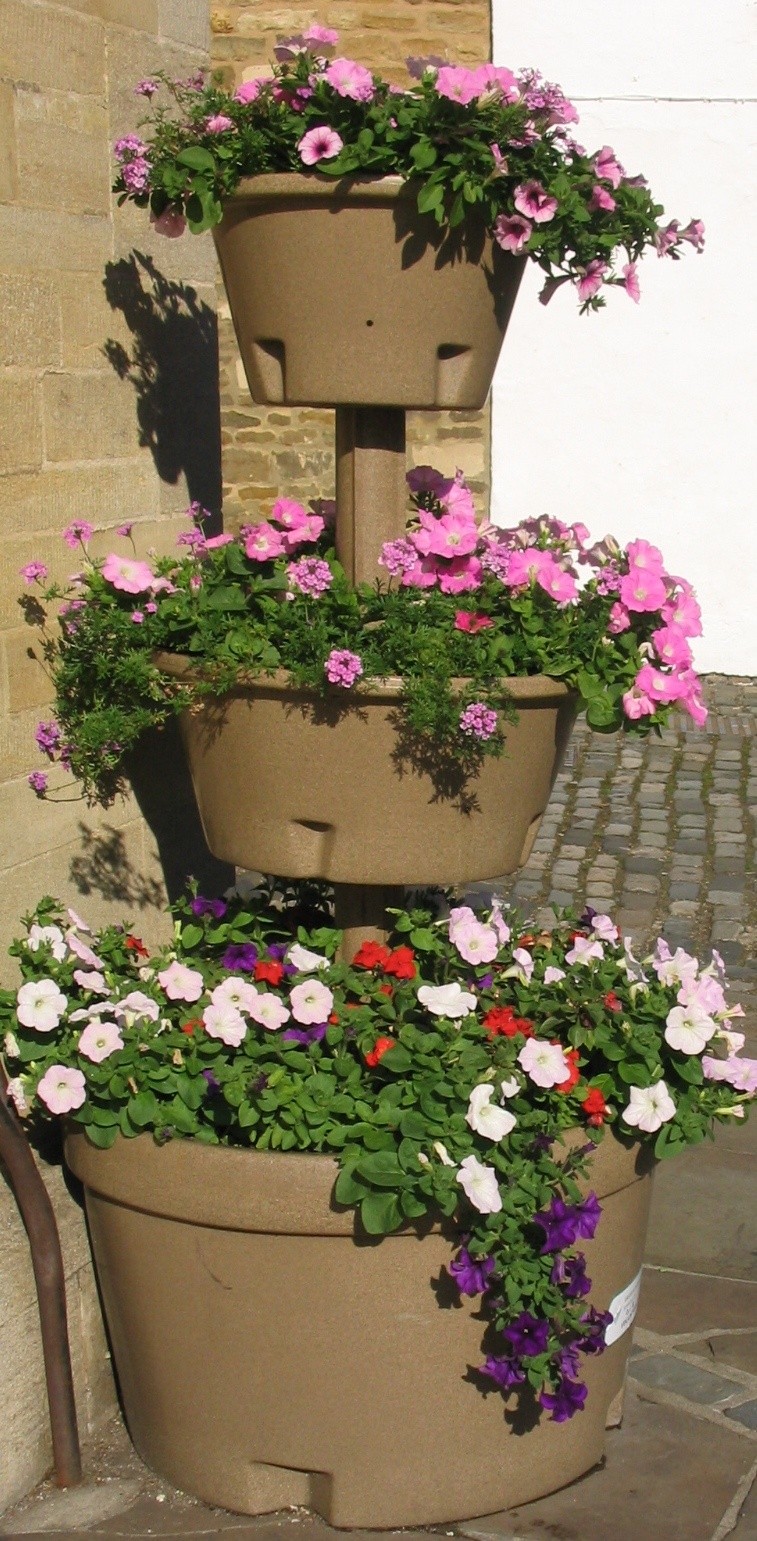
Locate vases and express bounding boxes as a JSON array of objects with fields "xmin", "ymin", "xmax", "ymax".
[
  {"xmin": 61, "ymin": 1114, "xmax": 661, "ymax": 1530},
  {"xmin": 153, "ymin": 652, "xmax": 580, "ymax": 884},
  {"xmin": 212, "ymin": 174, "xmax": 528, "ymax": 411}
]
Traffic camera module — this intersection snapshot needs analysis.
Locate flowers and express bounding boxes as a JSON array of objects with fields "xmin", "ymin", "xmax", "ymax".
[
  {"xmin": 1, "ymin": 861, "xmax": 757, "ymax": 1423},
  {"xmin": 111, "ymin": 26, "xmax": 706, "ymax": 316},
  {"xmin": 18, "ymin": 465, "xmax": 709, "ymax": 810}
]
